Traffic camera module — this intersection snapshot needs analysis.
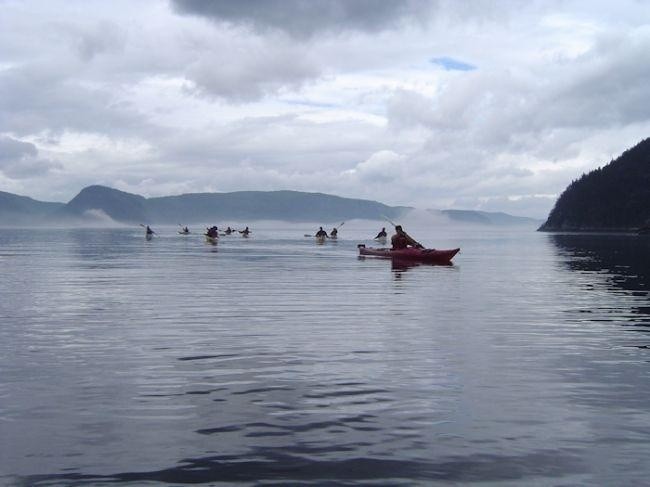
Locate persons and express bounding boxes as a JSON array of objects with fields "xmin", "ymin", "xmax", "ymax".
[
  {"xmin": 316, "ymin": 226, "xmax": 328, "ymax": 237},
  {"xmin": 182, "ymin": 225, "xmax": 248, "ymax": 237},
  {"xmin": 376, "ymin": 227, "xmax": 387, "ymax": 237},
  {"xmin": 330, "ymin": 227, "xmax": 337, "ymax": 235},
  {"xmin": 391, "ymin": 225, "xmax": 416, "ymax": 249},
  {"xmin": 147, "ymin": 225, "xmax": 152, "ymax": 233}
]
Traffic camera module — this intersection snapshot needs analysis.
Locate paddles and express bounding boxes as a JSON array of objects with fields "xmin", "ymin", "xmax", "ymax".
[
  {"xmin": 140, "ymin": 224, "xmax": 157, "ymax": 235},
  {"xmin": 378, "ymin": 213, "xmax": 422, "ymax": 248},
  {"xmin": 304, "ymin": 234, "xmax": 318, "ymax": 237},
  {"xmin": 336, "ymin": 222, "xmax": 344, "ymax": 230}
]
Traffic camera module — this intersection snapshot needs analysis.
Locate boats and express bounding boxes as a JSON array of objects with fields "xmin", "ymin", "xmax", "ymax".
[
  {"xmin": 178, "ymin": 229, "xmax": 251, "ymax": 243},
  {"xmin": 315, "ymin": 235, "xmax": 338, "ymax": 243},
  {"xmin": 357, "ymin": 234, "xmax": 461, "ymax": 262},
  {"xmin": 144, "ymin": 234, "xmax": 153, "ymax": 240}
]
